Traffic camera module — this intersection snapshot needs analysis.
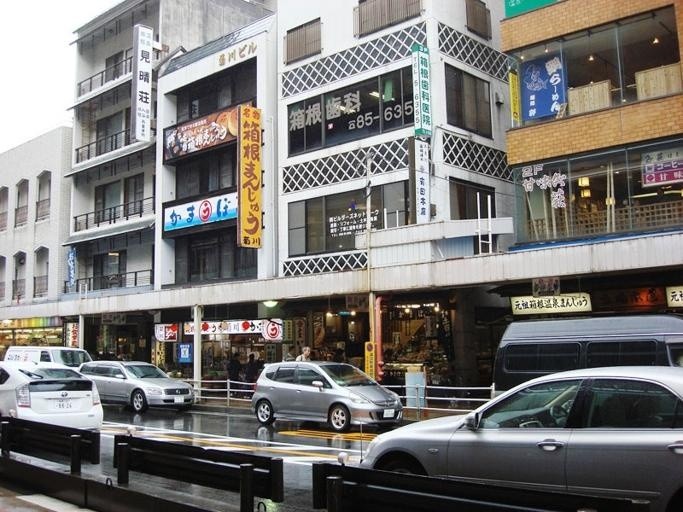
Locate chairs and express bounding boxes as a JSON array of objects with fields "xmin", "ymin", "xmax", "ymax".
[{"xmin": 596, "ymin": 394, "xmax": 660, "ymax": 428}]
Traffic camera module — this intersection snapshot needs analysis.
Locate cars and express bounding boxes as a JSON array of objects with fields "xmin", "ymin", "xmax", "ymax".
[
  {"xmin": 357, "ymin": 366, "xmax": 683, "ymax": 511},
  {"xmin": 77, "ymin": 359, "xmax": 195, "ymax": 412},
  {"xmin": 0, "ymin": 360, "xmax": 103, "ymax": 431},
  {"xmin": 251, "ymin": 359, "xmax": 403, "ymax": 431}
]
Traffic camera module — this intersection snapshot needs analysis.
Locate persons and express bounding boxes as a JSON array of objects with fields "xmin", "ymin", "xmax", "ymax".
[{"xmin": 200, "ymin": 324, "xmax": 438, "ymax": 400}]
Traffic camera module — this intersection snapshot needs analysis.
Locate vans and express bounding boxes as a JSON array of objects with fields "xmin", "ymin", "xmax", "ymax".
[
  {"xmin": 489, "ymin": 315, "xmax": 682, "ymax": 398},
  {"xmin": 2, "ymin": 346, "xmax": 92, "ymax": 372}
]
